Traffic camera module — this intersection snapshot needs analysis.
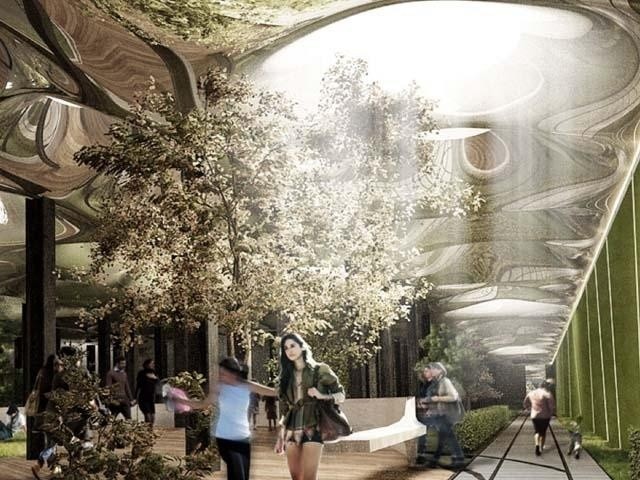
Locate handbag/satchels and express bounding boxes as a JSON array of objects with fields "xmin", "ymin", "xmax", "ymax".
[
  {"xmin": 447, "ymin": 401, "xmax": 465, "ymax": 422},
  {"xmin": 24, "ymin": 390, "xmax": 42, "ymax": 417},
  {"xmin": 317, "ymin": 398, "xmax": 353, "ymax": 440}
]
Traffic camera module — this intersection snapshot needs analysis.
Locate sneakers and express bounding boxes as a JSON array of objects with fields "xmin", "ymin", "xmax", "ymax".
[{"xmin": 32, "ymin": 465, "xmax": 42, "ymax": 479}]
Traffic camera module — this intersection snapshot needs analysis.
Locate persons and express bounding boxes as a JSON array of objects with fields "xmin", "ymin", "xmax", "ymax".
[
  {"xmin": 273, "ymin": 329, "xmax": 347, "ymax": 480},
  {"xmin": 264, "ymin": 383, "xmax": 280, "ymax": 429},
  {"xmin": 165, "ymin": 357, "xmax": 280, "ymax": 480},
  {"xmin": 557, "ymin": 415, "xmax": 584, "ymax": 460},
  {"xmin": 1, "ymin": 345, "xmax": 210, "ymax": 479},
  {"xmin": 248, "ymin": 378, "xmax": 261, "ymax": 431},
  {"xmin": 523, "ymin": 378, "xmax": 559, "ymax": 455},
  {"xmin": 414, "ymin": 364, "xmax": 440, "ymax": 465},
  {"xmin": 419, "ymin": 361, "xmax": 468, "ymax": 469}
]
